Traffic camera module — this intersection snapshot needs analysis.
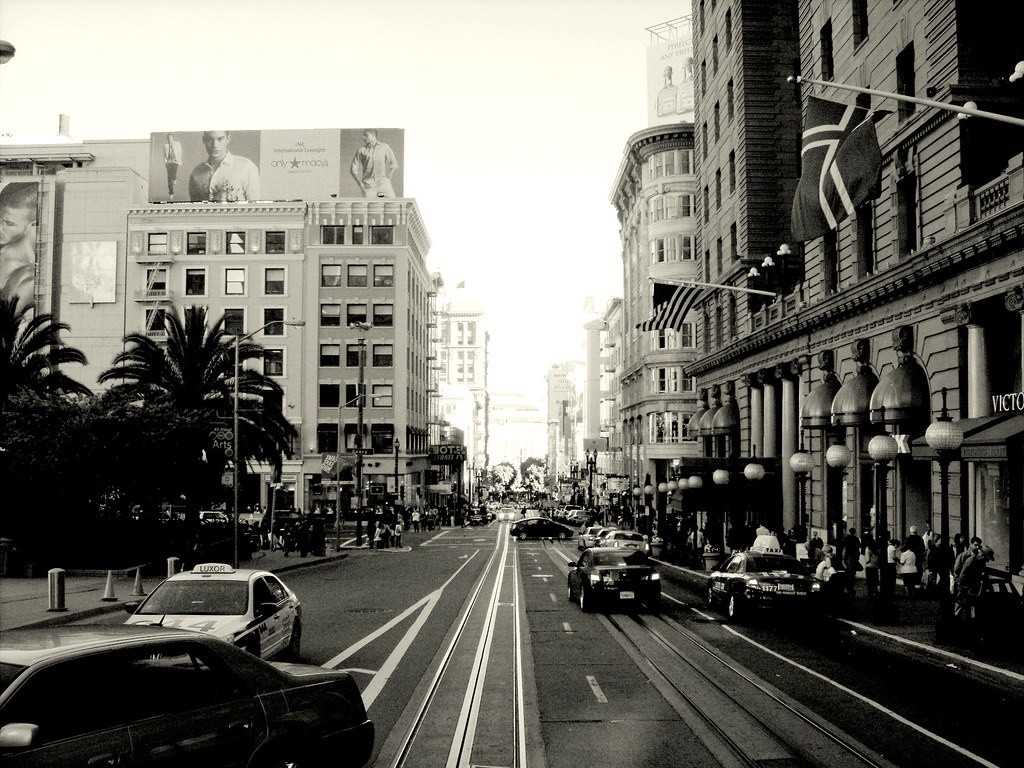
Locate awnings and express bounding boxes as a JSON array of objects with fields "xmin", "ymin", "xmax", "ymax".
[{"xmin": 911, "ymin": 414, "xmax": 1024, "ymax": 461}]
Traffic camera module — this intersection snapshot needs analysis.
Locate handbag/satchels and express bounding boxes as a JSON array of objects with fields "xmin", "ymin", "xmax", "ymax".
[{"xmin": 396, "ymin": 527, "xmax": 402, "ymax": 533}]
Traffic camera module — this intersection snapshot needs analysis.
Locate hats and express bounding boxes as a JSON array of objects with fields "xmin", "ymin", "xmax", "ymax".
[
  {"xmin": 909, "ymin": 525, "xmax": 918, "ymax": 533},
  {"xmin": 978, "ymin": 545, "xmax": 996, "ymax": 562}
]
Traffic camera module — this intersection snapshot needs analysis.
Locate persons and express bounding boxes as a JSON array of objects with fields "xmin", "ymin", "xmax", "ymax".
[
  {"xmin": 520, "ymin": 501, "xmax": 998, "ymax": 647},
  {"xmin": 162, "ymin": 131, "xmax": 260, "ymax": 201},
  {"xmin": 0, "ymin": 182, "xmax": 35, "ymax": 357},
  {"xmin": 369, "ymin": 502, "xmax": 487, "ymax": 551},
  {"xmin": 348, "ymin": 127, "xmax": 397, "ymax": 200}
]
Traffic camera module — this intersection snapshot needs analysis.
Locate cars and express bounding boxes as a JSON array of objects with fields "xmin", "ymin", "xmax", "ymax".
[
  {"xmin": 597, "ymin": 530, "xmax": 651, "ymax": 556},
  {"xmin": 548, "ymin": 503, "xmax": 595, "ymax": 524},
  {"xmin": 578, "ymin": 526, "xmax": 605, "ymax": 550},
  {"xmin": 707, "ymin": 551, "xmax": 826, "ymax": 629},
  {"xmin": 589, "ymin": 527, "xmax": 618, "ymax": 548},
  {"xmin": 120, "ymin": 563, "xmax": 305, "ymax": 664},
  {"xmin": 567, "ymin": 547, "xmax": 663, "ymax": 613},
  {"xmin": 510, "ymin": 517, "xmax": 574, "ymax": 540},
  {"xmin": 0, "ymin": 624, "xmax": 376, "ymax": 768}
]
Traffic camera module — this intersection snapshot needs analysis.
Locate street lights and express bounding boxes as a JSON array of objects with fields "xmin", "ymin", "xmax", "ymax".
[
  {"xmin": 790, "ymin": 427, "xmax": 815, "ymax": 539},
  {"xmin": 335, "ymin": 393, "xmax": 383, "ymax": 553},
  {"xmin": 826, "ymin": 416, "xmax": 851, "ymax": 599},
  {"xmin": 713, "ymin": 456, "xmax": 732, "ymax": 549},
  {"xmin": 585, "ymin": 447, "xmax": 598, "ymax": 511},
  {"xmin": 610, "ymin": 459, "xmax": 704, "ymax": 556},
  {"xmin": 745, "ymin": 441, "xmax": 765, "ymax": 546},
  {"xmin": 866, "ymin": 404, "xmax": 898, "ymax": 613},
  {"xmin": 234, "ymin": 320, "xmax": 307, "ymax": 569},
  {"xmin": 925, "ymin": 387, "xmax": 963, "ymax": 615}
]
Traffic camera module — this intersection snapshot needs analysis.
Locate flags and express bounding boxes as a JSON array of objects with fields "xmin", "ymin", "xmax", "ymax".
[
  {"xmin": 635, "ymin": 281, "xmax": 704, "ymax": 332},
  {"xmin": 791, "ymin": 96, "xmax": 895, "ymax": 243}
]
